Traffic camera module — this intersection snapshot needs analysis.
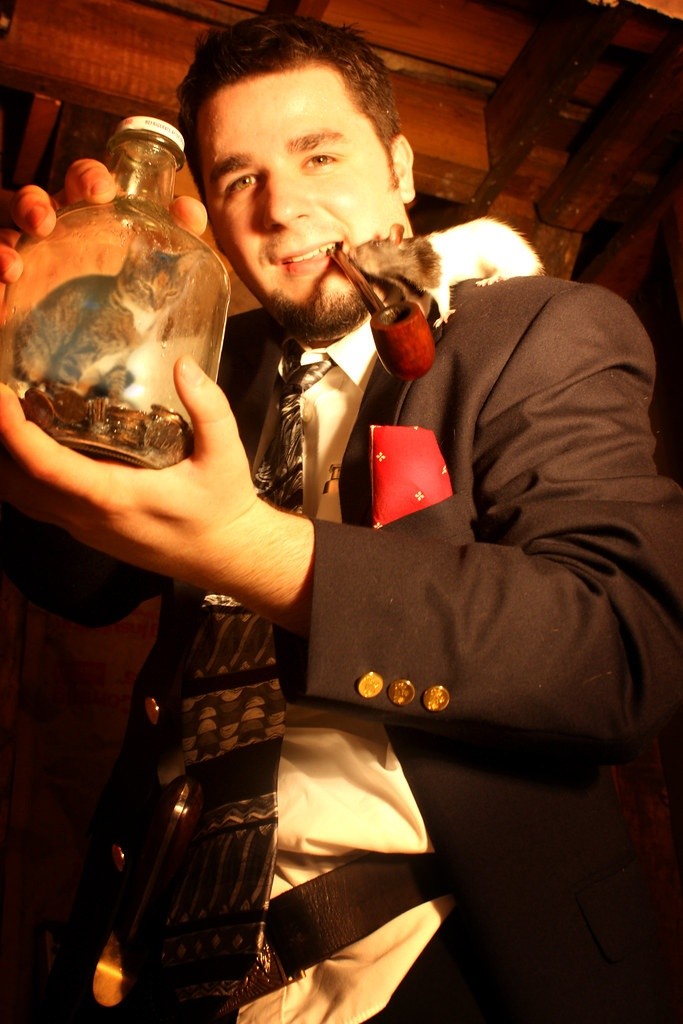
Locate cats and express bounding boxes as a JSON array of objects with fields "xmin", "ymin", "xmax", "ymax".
[{"xmin": 10, "ymin": 234, "xmax": 205, "ymax": 409}]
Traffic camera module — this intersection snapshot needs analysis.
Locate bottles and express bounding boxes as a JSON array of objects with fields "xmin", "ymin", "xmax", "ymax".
[{"xmin": 0, "ymin": 116, "xmax": 233, "ymax": 471}]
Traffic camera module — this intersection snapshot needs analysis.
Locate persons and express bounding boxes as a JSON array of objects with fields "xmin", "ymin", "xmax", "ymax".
[{"xmin": 0, "ymin": 11, "xmax": 683, "ymax": 1024}]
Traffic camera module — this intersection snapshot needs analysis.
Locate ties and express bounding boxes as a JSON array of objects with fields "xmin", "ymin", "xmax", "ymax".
[{"xmin": 124, "ymin": 346, "xmax": 332, "ymax": 1024}]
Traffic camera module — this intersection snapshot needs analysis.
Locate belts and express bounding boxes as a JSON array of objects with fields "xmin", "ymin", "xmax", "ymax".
[{"xmin": 236, "ymin": 854, "xmax": 453, "ymax": 1006}]
